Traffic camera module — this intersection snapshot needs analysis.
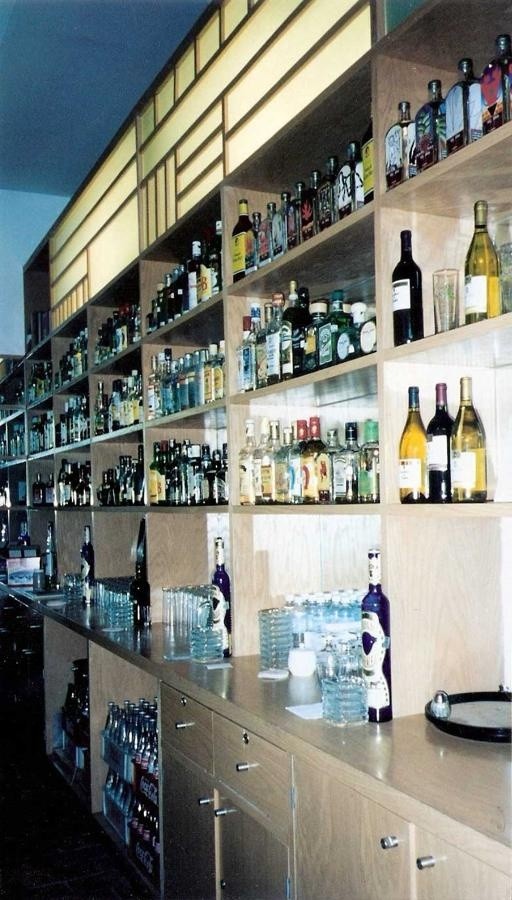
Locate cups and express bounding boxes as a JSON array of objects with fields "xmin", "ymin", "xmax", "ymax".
[
  {"xmin": 93, "ymin": 575, "xmax": 134, "ymax": 636},
  {"xmin": 61, "ymin": 570, "xmax": 82, "ymax": 611},
  {"xmin": 258, "ymin": 598, "xmax": 367, "ymax": 730},
  {"xmin": 213, "ymin": 628, "xmax": 225, "ymax": 658},
  {"xmin": 160, "ymin": 582, "xmax": 212, "ymax": 666}
]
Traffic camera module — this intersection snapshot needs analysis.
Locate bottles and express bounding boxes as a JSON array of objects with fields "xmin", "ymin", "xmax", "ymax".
[
  {"xmin": 479, "ymin": 34, "xmax": 511, "ymax": 135},
  {"xmin": 426, "ymin": 383, "xmax": 455, "ymax": 503},
  {"xmin": 212, "ymin": 537, "xmax": 231, "ymax": 658},
  {"xmin": 1, "ymin": 514, "xmax": 59, "ymax": 596},
  {"xmin": 0, "ymin": 478, "xmax": 11, "ymax": 507},
  {"xmin": 95, "ymin": 441, "xmax": 145, "ymax": 507},
  {"xmin": 27, "ymin": 358, "xmax": 53, "ymax": 405},
  {"xmin": 414, "ymin": 79, "xmax": 447, "ymax": 176},
  {"xmin": 234, "ymin": 408, "xmax": 378, "ymax": 507},
  {"xmin": 397, "ymin": 386, "xmax": 426, "ymax": 504},
  {"xmin": 383, "ymin": 101, "xmax": 416, "ymax": 191},
  {"xmin": 59, "ymin": 657, "xmax": 91, "ymax": 797},
  {"xmin": 29, "ymin": 409, "xmax": 55, "ymax": 457},
  {"xmin": 54, "ymin": 326, "xmax": 89, "ymax": 390},
  {"xmin": 235, "ymin": 278, "xmax": 376, "ymax": 392},
  {"xmin": 98, "ymin": 695, "xmax": 161, "ymax": 897},
  {"xmin": 15, "ymin": 467, "xmax": 26, "ymax": 507},
  {"xmin": 449, "ymin": 377, "xmax": 487, "ymax": 503},
  {"xmin": 135, "ymin": 562, "xmax": 151, "ymax": 629},
  {"xmin": 462, "ymin": 200, "xmax": 503, "ymax": 328},
  {"xmin": 56, "ymin": 457, "xmax": 90, "ymax": 508},
  {"xmin": 0, "ymin": 598, "xmax": 45, "ymax": 741},
  {"xmin": 145, "ymin": 220, "xmax": 223, "ymax": 336},
  {"xmin": 1, "ymin": 362, "xmax": 25, "ymax": 421},
  {"xmin": 81, "ymin": 526, "xmax": 93, "ymax": 609},
  {"xmin": 391, "ymin": 230, "xmax": 424, "ymax": 347},
  {"xmin": 0, "ymin": 435, "xmax": 8, "ymax": 465},
  {"xmin": 361, "ymin": 548, "xmax": 391, "ymax": 723},
  {"xmin": 9, "ymin": 424, "xmax": 26, "ymax": 459},
  {"xmin": 146, "ymin": 339, "xmax": 225, "ymax": 422},
  {"xmin": 93, "ymin": 370, "xmax": 145, "ymax": 437},
  {"xmin": 444, "ymin": 58, "xmax": 483, "ymax": 156},
  {"xmin": 94, "ymin": 301, "xmax": 141, "ymax": 367},
  {"xmin": 229, "ymin": 97, "xmax": 375, "ymax": 284},
  {"xmin": 145, "ymin": 436, "xmax": 230, "ymax": 507},
  {"xmin": 55, "ymin": 392, "xmax": 90, "ymax": 447}
]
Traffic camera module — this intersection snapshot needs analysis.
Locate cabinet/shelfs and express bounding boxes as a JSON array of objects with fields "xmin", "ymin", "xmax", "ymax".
[
  {"xmin": 154, "ymin": 677, "xmax": 297, "ymax": 900},
  {"xmin": 292, "ymin": 747, "xmax": 510, "ymax": 900},
  {"xmin": 0, "ymin": 0, "xmax": 511, "ymax": 723},
  {"xmin": 0, "ymin": 589, "xmax": 87, "ymax": 755}
]
